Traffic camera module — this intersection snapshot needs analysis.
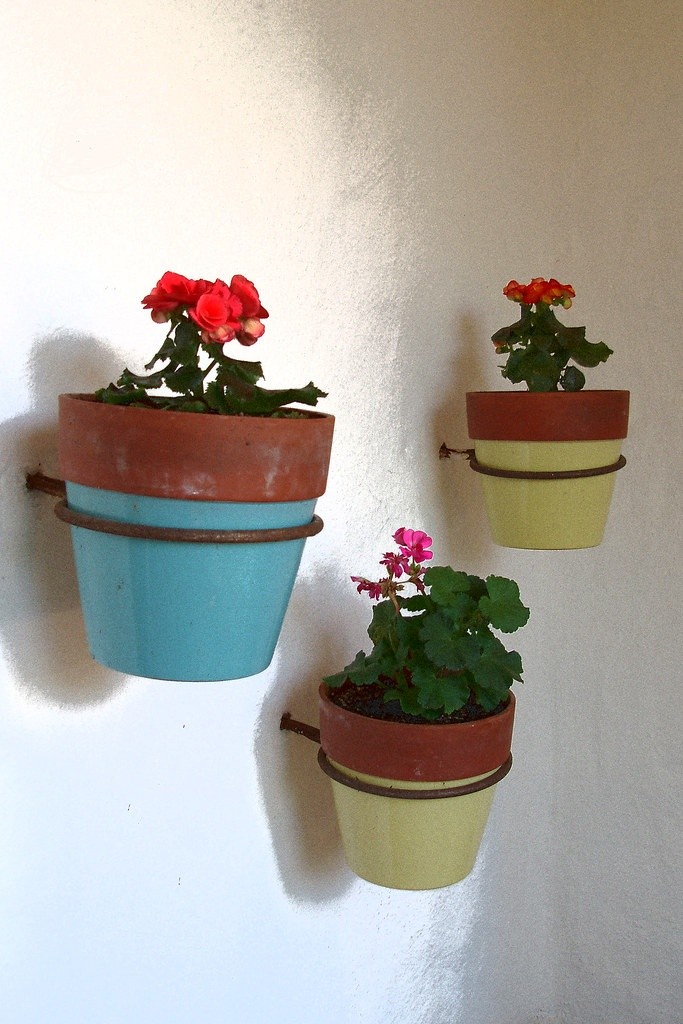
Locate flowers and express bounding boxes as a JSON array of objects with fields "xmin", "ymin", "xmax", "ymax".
[
  {"xmin": 325, "ymin": 528, "xmax": 530, "ymax": 720},
  {"xmin": 491, "ymin": 279, "xmax": 614, "ymax": 391},
  {"xmin": 97, "ymin": 272, "xmax": 327, "ymax": 414}
]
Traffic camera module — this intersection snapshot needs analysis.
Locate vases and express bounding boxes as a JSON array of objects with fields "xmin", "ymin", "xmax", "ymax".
[
  {"xmin": 442, "ymin": 389, "xmax": 632, "ymax": 546},
  {"xmin": 279, "ymin": 680, "xmax": 515, "ymax": 890},
  {"xmin": 29, "ymin": 387, "xmax": 335, "ymax": 681}
]
